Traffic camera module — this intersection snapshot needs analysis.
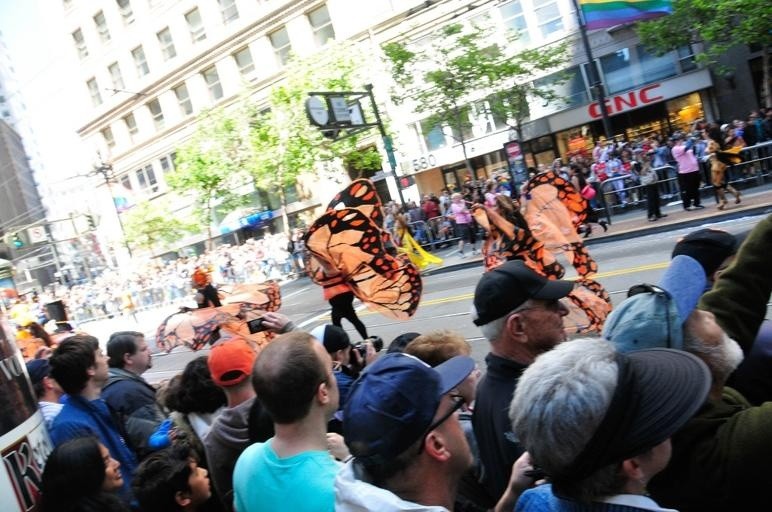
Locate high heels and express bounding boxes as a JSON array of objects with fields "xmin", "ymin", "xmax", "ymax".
[
  {"xmin": 599, "ymin": 220, "xmax": 608, "ymax": 233},
  {"xmin": 583, "ymin": 227, "xmax": 592, "ymax": 238}
]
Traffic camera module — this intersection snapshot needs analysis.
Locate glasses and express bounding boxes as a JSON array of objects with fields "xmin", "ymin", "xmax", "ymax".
[
  {"xmin": 626, "ymin": 282, "xmax": 674, "ymax": 350},
  {"xmin": 417, "ymin": 394, "xmax": 466, "ymax": 456}
]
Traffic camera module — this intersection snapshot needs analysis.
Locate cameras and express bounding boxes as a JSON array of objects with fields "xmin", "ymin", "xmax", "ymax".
[{"xmin": 350, "ymin": 335, "xmax": 383, "ymax": 360}]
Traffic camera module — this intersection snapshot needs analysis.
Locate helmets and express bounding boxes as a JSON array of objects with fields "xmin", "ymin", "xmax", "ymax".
[{"xmin": 670, "ymin": 133, "xmax": 684, "ymax": 141}]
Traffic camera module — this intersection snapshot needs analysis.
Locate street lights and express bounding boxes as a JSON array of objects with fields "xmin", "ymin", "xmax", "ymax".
[{"xmin": 86, "ymin": 148, "xmax": 136, "ymax": 262}]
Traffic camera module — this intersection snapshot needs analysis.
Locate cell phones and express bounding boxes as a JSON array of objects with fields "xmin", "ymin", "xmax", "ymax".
[{"xmin": 247, "ymin": 317, "xmax": 267, "ymax": 336}]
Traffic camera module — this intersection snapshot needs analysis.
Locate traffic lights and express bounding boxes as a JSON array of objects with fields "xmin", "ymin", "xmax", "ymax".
[
  {"xmin": 86, "ymin": 214, "xmax": 94, "ymax": 229},
  {"xmin": 12, "ymin": 232, "xmax": 23, "ymax": 248}
]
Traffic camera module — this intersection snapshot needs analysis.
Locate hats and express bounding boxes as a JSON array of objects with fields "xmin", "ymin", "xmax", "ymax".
[
  {"xmin": 671, "ymin": 228, "xmax": 738, "ymax": 279},
  {"xmin": 310, "ymin": 323, "xmax": 355, "ymax": 354},
  {"xmin": 341, "ymin": 350, "xmax": 475, "ymax": 456},
  {"xmin": 207, "ymin": 336, "xmax": 257, "ymax": 387},
  {"xmin": 526, "ymin": 343, "xmax": 714, "ymax": 484},
  {"xmin": 471, "ymin": 259, "xmax": 576, "ymax": 328},
  {"xmin": 597, "ymin": 254, "xmax": 709, "ymax": 354},
  {"xmin": 26, "ymin": 358, "xmax": 49, "ymax": 384}
]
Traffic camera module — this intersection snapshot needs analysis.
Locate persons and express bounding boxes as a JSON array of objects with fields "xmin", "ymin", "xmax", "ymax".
[
  {"xmin": 306, "ymin": 177, "xmax": 422, "ymax": 340},
  {"xmin": 323, "ymin": 325, "xmax": 378, "ymax": 421},
  {"xmin": 508, "ymin": 338, "xmax": 714, "ymax": 511},
  {"xmin": 407, "ymin": 329, "xmax": 481, "ymax": 461},
  {"xmin": 471, "ymin": 172, "xmax": 612, "ymax": 335},
  {"xmin": 174, "ymin": 355, "xmax": 229, "ymax": 466},
  {"xmin": 334, "ymin": 354, "xmax": 545, "ymax": 512},
  {"xmin": 671, "ymin": 228, "xmax": 738, "ymax": 293},
  {"xmin": 25, "ymin": 359, "xmax": 66, "ymax": 433},
  {"xmin": 35, "ymin": 437, "xmax": 133, "ymax": 510},
  {"xmin": 2, "ymin": 254, "xmax": 195, "ymax": 346},
  {"xmin": 194, "ymin": 250, "xmax": 222, "ymax": 265},
  {"xmin": 220, "ymin": 230, "xmax": 306, "ymax": 286},
  {"xmin": 380, "ymin": 177, "xmax": 502, "ymax": 253},
  {"xmin": 46, "ymin": 333, "xmax": 138, "ymax": 507},
  {"xmin": 190, "ymin": 266, "xmax": 222, "ymax": 308},
  {"xmin": 387, "ymin": 332, "xmax": 421, "ymax": 351},
  {"xmin": 470, "ymin": 261, "xmax": 576, "ymax": 511},
  {"xmin": 552, "ymin": 107, "xmax": 772, "ymax": 236},
  {"xmin": 99, "ymin": 332, "xmax": 158, "ymax": 434},
  {"xmin": 231, "ymin": 332, "xmax": 345, "ymax": 512},
  {"xmin": 134, "ymin": 446, "xmax": 212, "ymax": 511},
  {"xmin": 601, "ymin": 213, "xmax": 770, "ymax": 511},
  {"xmin": 148, "ymin": 375, "xmax": 183, "ymax": 447},
  {"xmin": 203, "ymin": 312, "xmax": 296, "ymax": 512}
]
